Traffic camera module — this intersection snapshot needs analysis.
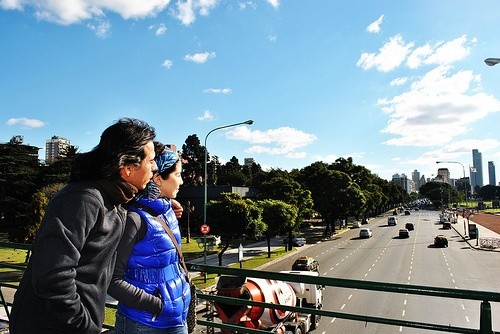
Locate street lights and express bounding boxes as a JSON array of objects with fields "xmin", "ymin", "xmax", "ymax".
[
  {"xmin": 436, "ymin": 160, "xmax": 469, "ymax": 226},
  {"xmin": 183, "ymin": 201, "xmax": 195, "ymax": 244},
  {"xmin": 202, "ymin": 120, "xmax": 255, "ymax": 278}
]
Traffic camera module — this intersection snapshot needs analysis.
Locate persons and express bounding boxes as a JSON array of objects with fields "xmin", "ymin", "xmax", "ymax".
[
  {"xmin": 9, "ymin": 117, "xmax": 184, "ymax": 334},
  {"xmin": 107, "ymin": 141, "xmax": 191, "ymax": 334}
]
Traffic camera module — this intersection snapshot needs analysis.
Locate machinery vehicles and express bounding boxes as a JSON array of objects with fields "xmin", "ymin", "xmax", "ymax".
[{"xmin": 212, "ymin": 271, "xmax": 326, "ymax": 334}]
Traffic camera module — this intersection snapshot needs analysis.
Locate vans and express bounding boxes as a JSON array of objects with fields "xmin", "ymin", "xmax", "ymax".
[{"xmin": 388, "ymin": 216, "xmax": 399, "ymax": 225}]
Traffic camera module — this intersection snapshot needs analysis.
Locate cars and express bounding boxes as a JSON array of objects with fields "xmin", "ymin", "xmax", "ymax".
[
  {"xmin": 434, "ymin": 235, "xmax": 448, "ymax": 248},
  {"xmin": 399, "ymin": 229, "xmax": 410, "ymax": 238},
  {"xmin": 362, "ymin": 219, "xmax": 369, "ymax": 224},
  {"xmin": 284, "ymin": 234, "xmax": 306, "ymax": 247},
  {"xmin": 353, "ymin": 222, "xmax": 361, "ymax": 228},
  {"xmin": 438, "ymin": 208, "xmax": 460, "ymax": 225},
  {"xmin": 291, "ymin": 257, "xmax": 320, "ymax": 273},
  {"xmin": 360, "ymin": 229, "xmax": 372, "ymax": 239},
  {"xmin": 393, "ymin": 199, "xmax": 433, "ymax": 216}
]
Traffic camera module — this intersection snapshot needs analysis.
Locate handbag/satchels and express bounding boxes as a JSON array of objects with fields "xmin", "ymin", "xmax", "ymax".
[{"xmin": 188, "ymin": 278, "xmax": 196, "ymax": 333}]
